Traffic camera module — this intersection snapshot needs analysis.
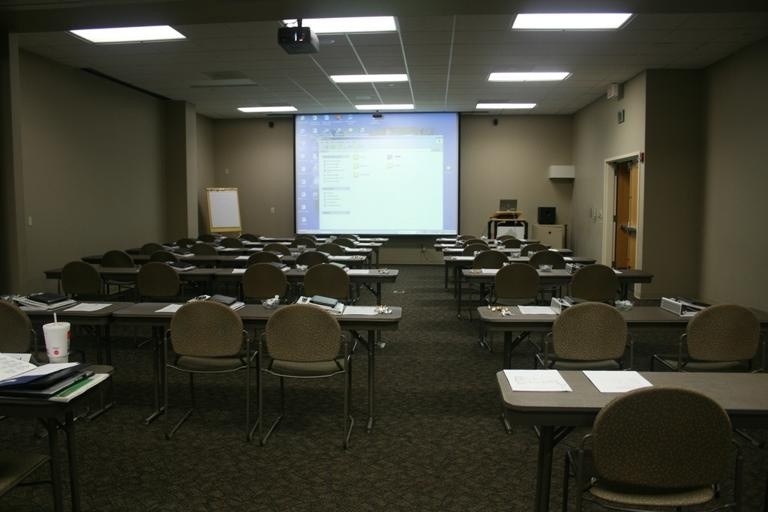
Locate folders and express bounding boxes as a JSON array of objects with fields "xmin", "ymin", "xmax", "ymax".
[
  {"xmin": 296, "ymin": 296, "xmax": 345, "ymax": 316},
  {"xmin": 565, "ymin": 263, "xmax": 580, "ymax": 274},
  {"xmin": 660, "ymin": 297, "xmax": 706, "ymax": 317},
  {"xmin": 186, "ymin": 294, "xmax": 246, "ymax": 313},
  {"xmin": 12, "ymin": 292, "xmax": 78, "ymax": 312},
  {"xmin": 550, "ymin": 297, "xmax": 569, "ymax": 315}
]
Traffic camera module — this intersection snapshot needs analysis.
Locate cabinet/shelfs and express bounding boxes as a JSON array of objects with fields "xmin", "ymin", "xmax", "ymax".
[{"xmin": 533, "ymin": 224, "xmax": 566, "ymax": 249}]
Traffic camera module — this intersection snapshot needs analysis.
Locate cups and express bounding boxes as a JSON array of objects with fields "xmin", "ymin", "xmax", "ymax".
[{"xmin": 42, "ymin": 322, "xmax": 71, "ymax": 358}]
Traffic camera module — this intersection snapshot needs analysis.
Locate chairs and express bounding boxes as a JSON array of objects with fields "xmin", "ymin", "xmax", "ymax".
[
  {"xmin": 243, "ymin": 266, "xmax": 291, "ymax": 344},
  {"xmin": 304, "ymin": 265, "xmax": 358, "ymax": 353},
  {"xmin": 162, "ymin": 301, "xmax": 258, "ymax": 441},
  {"xmin": 293, "ymin": 251, "xmax": 330, "ymax": 269},
  {"xmin": 219, "ymin": 238, "xmax": 245, "ymax": 248},
  {"xmin": 263, "ymin": 244, "xmax": 290, "ymax": 255},
  {"xmin": 239, "ymin": 233, "xmax": 260, "ymax": 242},
  {"xmin": 290, "ymin": 238, "xmax": 316, "ymax": 247},
  {"xmin": 296, "ymin": 234, "xmax": 317, "ymax": 242},
  {"xmin": 480, "ymin": 262, "xmax": 545, "ymax": 353},
  {"xmin": 247, "ymin": 251, "xmax": 282, "ymax": 268},
  {"xmin": 496, "ymin": 235, "xmax": 517, "ymax": 240},
  {"xmin": 257, "ymin": 304, "xmax": 357, "ymax": 448},
  {"xmin": 563, "ymin": 388, "xmax": 745, "ymax": 512},
  {"xmin": 462, "ymin": 244, "xmax": 491, "ymax": 256},
  {"xmin": 650, "ymin": 305, "xmax": 761, "ymax": 373},
  {"xmin": 338, "ymin": 235, "xmax": 359, "ymax": 242},
  {"xmin": 520, "ymin": 244, "xmax": 549, "ymax": 256},
  {"xmin": 100, "ymin": 250, "xmax": 139, "ymax": 295},
  {"xmin": 141, "ymin": 243, "xmax": 166, "ymax": 255},
  {"xmin": 332, "ymin": 238, "xmax": 356, "ymax": 249},
  {"xmin": 501, "ymin": 239, "xmax": 525, "ymax": 249},
  {"xmin": 316, "ymin": 244, "xmax": 343, "ymax": 255},
  {"xmin": 469, "ymin": 251, "xmax": 510, "ymax": 323},
  {"xmin": 176, "ymin": 238, "xmax": 195, "ymax": 246},
  {"xmin": 191, "ymin": 244, "xmax": 217, "ymax": 255},
  {"xmin": 463, "ymin": 239, "xmax": 490, "ymax": 248},
  {"xmin": 457, "ymin": 235, "xmax": 481, "ymax": 241},
  {"xmin": 151, "ymin": 252, "xmax": 178, "ymax": 264},
  {"xmin": 199, "ymin": 234, "xmax": 222, "ymax": 242},
  {"xmin": 533, "ymin": 304, "xmax": 633, "ymax": 438},
  {"xmin": 61, "ymin": 260, "xmax": 102, "ymax": 301},
  {"xmin": 571, "ymin": 264, "xmax": 622, "ymax": 303},
  {"xmin": 529, "ymin": 252, "xmax": 565, "ymax": 305},
  {"xmin": 136, "ymin": 262, "xmax": 186, "ymax": 302},
  {"xmin": 0, "ymin": 302, "xmax": 86, "ymax": 440}
]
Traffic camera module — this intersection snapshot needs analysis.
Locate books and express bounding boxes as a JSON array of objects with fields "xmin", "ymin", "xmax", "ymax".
[
  {"xmin": 27, "ymin": 293, "xmax": 69, "ymax": 304},
  {"xmin": 308, "ymin": 294, "xmax": 340, "ymax": 309},
  {"xmin": 563, "ymin": 296, "xmax": 589, "ymax": 305},
  {"xmin": 208, "ymin": 293, "xmax": 236, "ymax": 306}
]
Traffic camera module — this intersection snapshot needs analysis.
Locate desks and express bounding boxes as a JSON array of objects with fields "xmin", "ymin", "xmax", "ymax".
[
  {"xmin": 444, "ymin": 255, "xmax": 597, "ymax": 319},
  {"xmin": 497, "ymin": 370, "xmax": 768, "ymax": 512},
  {"xmin": 82, "ymin": 253, "xmax": 215, "ymax": 296},
  {"xmin": 196, "ymin": 237, "xmax": 295, "ymax": 242},
  {"xmin": 436, "ymin": 239, "xmax": 540, "ymax": 244},
  {"xmin": 43, "ymin": 267, "xmax": 222, "ymax": 302},
  {"xmin": 478, "ymin": 299, "xmax": 768, "ymax": 434},
  {"xmin": 125, "ymin": 247, "xmax": 244, "ymax": 254},
  {"xmin": 265, "ymin": 242, "xmax": 383, "ymax": 268},
  {"xmin": 284, "ymin": 237, "xmax": 389, "ymax": 268},
  {"xmin": 218, "ymin": 255, "xmax": 367, "ymax": 301},
  {"xmin": 0, "ymin": 364, "xmax": 114, "ymax": 512},
  {"xmin": 114, "ymin": 302, "xmax": 403, "ymax": 434},
  {"xmin": 441, "ymin": 247, "xmax": 575, "ymax": 289},
  {"xmin": 0, "ymin": 301, "xmax": 132, "ymax": 422},
  {"xmin": 248, "ymin": 247, "xmax": 398, "ymax": 303},
  {"xmin": 463, "ymin": 267, "xmax": 654, "ymax": 353},
  {"xmin": 164, "ymin": 241, "xmax": 267, "ymax": 248},
  {"xmin": 433, "ymin": 243, "xmax": 551, "ymax": 289},
  {"xmin": 213, "ymin": 268, "xmax": 401, "ymax": 350}
]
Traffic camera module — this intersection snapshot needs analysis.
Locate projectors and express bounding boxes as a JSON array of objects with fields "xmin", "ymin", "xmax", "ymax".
[{"xmin": 278, "ymin": 27, "xmax": 319, "ymax": 56}]
